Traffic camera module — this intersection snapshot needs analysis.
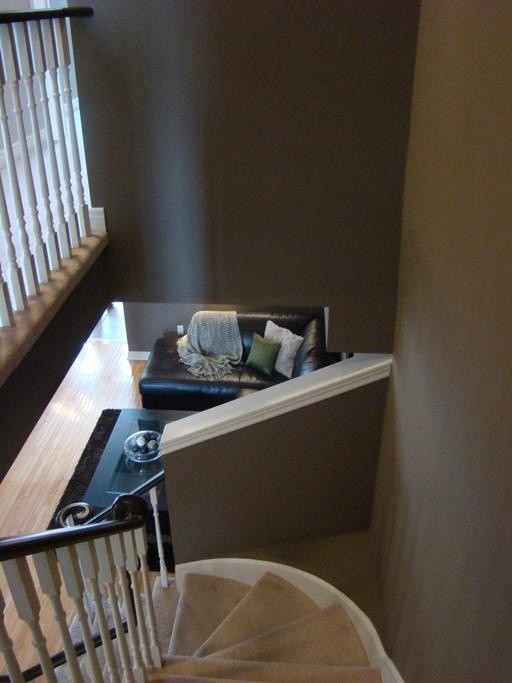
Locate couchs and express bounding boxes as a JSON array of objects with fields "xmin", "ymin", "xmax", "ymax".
[{"xmin": 139, "ymin": 311, "xmax": 336, "ymax": 410}]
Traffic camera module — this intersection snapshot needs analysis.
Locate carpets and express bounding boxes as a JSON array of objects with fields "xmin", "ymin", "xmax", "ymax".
[{"xmin": 44, "ymin": 407, "xmax": 175, "ymax": 575}]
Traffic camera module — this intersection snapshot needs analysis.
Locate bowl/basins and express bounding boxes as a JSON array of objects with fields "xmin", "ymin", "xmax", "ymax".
[{"xmin": 124, "ymin": 430, "xmax": 162, "ymax": 462}]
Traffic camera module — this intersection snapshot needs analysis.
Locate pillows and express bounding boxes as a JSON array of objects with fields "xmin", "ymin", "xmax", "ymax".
[{"xmin": 244, "ymin": 319, "xmax": 304, "ymax": 380}]
